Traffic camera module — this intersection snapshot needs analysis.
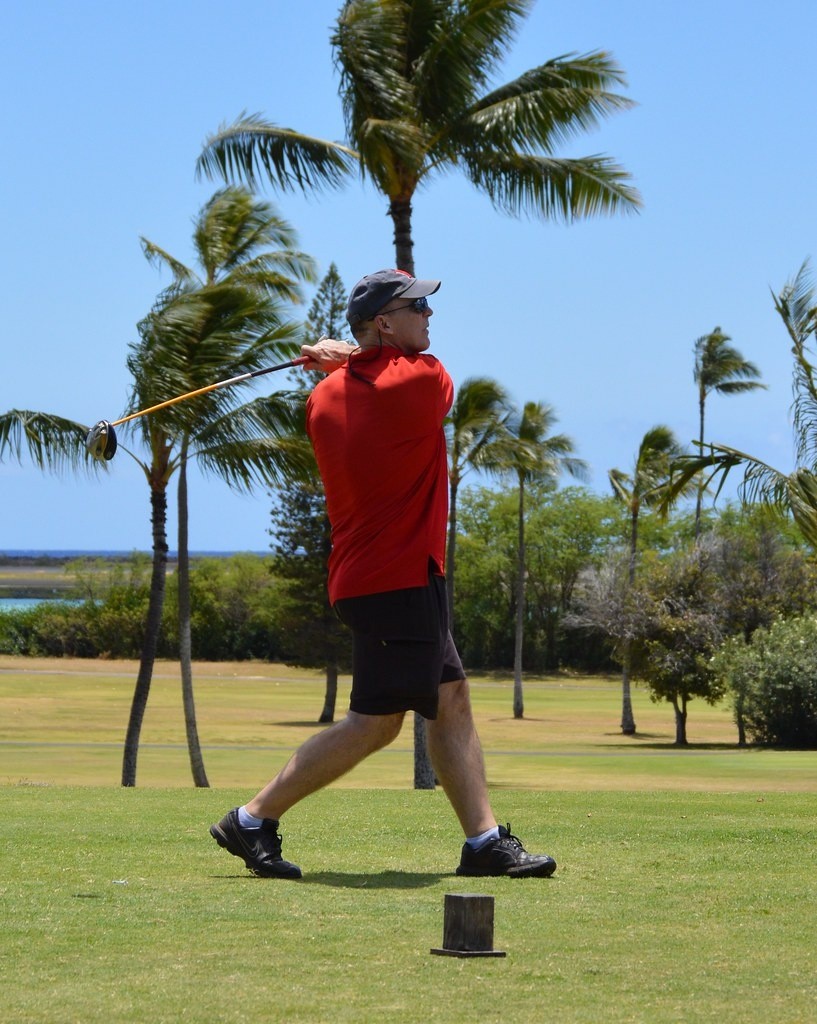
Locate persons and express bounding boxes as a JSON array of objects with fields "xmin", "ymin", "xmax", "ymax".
[{"xmin": 207, "ymin": 267, "xmax": 556, "ymax": 879}]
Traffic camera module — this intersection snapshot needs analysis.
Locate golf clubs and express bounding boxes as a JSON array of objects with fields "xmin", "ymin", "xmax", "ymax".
[{"xmin": 84, "ymin": 353, "xmax": 308, "ymax": 462}]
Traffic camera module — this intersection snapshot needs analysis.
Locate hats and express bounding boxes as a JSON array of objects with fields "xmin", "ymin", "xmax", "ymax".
[{"xmin": 347, "ymin": 268, "xmax": 442, "ymax": 325}]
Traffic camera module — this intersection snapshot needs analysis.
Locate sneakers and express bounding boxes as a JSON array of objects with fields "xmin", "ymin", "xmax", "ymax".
[
  {"xmin": 455, "ymin": 822, "xmax": 556, "ymax": 878},
  {"xmin": 209, "ymin": 808, "xmax": 302, "ymax": 880}
]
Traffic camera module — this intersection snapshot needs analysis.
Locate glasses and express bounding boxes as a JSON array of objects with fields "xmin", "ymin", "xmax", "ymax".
[{"xmin": 367, "ymin": 297, "xmax": 427, "ymax": 323}]
